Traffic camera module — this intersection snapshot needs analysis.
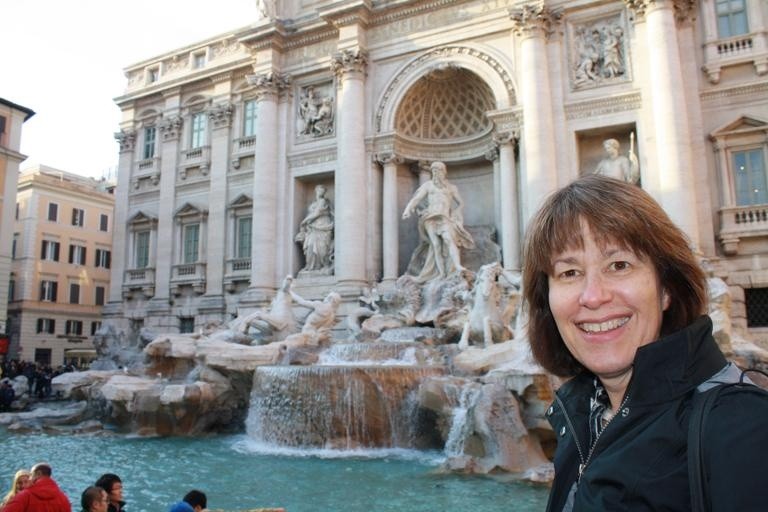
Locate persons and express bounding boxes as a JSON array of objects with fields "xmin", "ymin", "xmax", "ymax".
[
  {"xmin": 283, "ymin": 289, "xmax": 341, "ymax": 343},
  {"xmin": 600, "ymin": 27, "xmax": 621, "ymax": 80},
  {"xmin": 592, "ymin": 137, "xmax": 641, "ymax": 188},
  {"xmin": 314, "ymin": 95, "xmax": 332, "ymax": 135},
  {"xmin": 519, "ymin": 176, "xmax": 768, "ymax": 512},
  {"xmin": 300, "ymin": 183, "xmax": 334, "ymax": 272},
  {"xmin": 79, "ymin": 485, "xmax": 111, "ymax": 512},
  {"xmin": 96, "ymin": 473, "xmax": 127, "ymax": 512},
  {"xmin": 299, "ymin": 87, "xmax": 321, "ymax": 134},
  {"xmin": 3, "ymin": 463, "xmax": 71, "ymax": 512},
  {"xmin": 574, "ymin": 46, "xmax": 601, "ymax": 82},
  {"xmin": 184, "ymin": 489, "xmax": 209, "ymax": 512},
  {"xmin": 402, "ymin": 159, "xmax": 476, "ymax": 285},
  {"xmin": 0, "ymin": 469, "xmax": 30, "ymax": 512},
  {"xmin": 0, "ymin": 357, "xmax": 93, "ymax": 412}
]
